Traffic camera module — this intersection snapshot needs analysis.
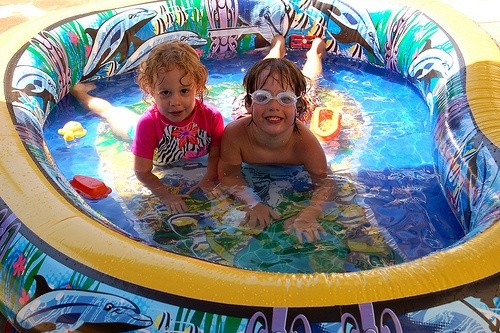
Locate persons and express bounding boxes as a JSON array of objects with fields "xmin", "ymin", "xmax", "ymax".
[
  {"xmin": 71, "ymin": 40, "xmax": 223, "ymax": 215},
  {"xmin": 217, "ymin": 35, "xmax": 337, "ymax": 243}
]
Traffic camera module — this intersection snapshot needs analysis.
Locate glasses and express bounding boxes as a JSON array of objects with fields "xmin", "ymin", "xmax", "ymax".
[{"xmin": 249, "ymin": 90, "xmax": 301, "ymax": 106}]
